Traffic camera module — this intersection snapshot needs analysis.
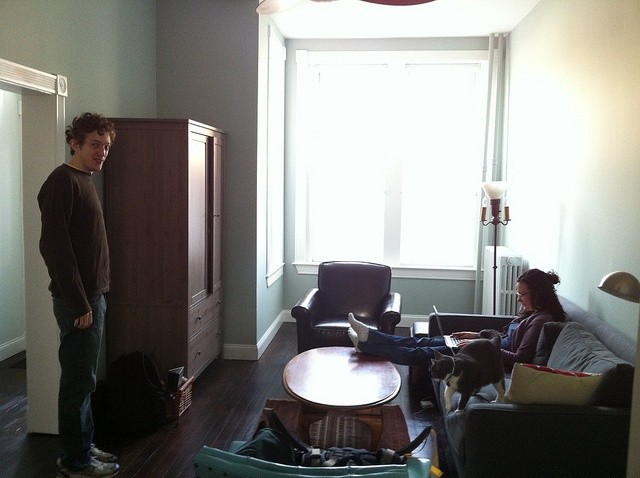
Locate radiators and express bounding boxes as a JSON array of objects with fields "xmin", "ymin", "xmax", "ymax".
[{"xmin": 483, "ymin": 246, "xmax": 523, "ymax": 316}]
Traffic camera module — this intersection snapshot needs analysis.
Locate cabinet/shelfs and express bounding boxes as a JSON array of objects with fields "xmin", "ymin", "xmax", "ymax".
[{"xmin": 101, "ymin": 118, "xmax": 225, "ymax": 387}]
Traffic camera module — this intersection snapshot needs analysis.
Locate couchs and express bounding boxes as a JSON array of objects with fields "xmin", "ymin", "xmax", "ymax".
[
  {"xmin": 428, "ymin": 311, "xmax": 634, "ymax": 478},
  {"xmin": 192, "ymin": 439, "xmax": 432, "ymax": 477},
  {"xmin": 289, "ymin": 261, "xmax": 401, "ymax": 355}
]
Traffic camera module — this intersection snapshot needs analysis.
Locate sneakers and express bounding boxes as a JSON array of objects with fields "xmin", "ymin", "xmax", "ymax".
[
  {"xmin": 87, "ymin": 443, "xmax": 117, "ymax": 464},
  {"xmin": 55, "ymin": 454, "xmax": 120, "ymax": 478}
]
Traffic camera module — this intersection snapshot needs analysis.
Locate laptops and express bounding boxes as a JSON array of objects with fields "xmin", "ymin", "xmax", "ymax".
[{"xmin": 433, "ymin": 304, "xmax": 464, "ymax": 350}]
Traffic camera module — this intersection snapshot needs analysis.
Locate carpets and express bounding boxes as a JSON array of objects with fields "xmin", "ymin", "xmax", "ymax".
[{"xmin": 254, "ymin": 399, "xmax": 412, "ymax": 454}]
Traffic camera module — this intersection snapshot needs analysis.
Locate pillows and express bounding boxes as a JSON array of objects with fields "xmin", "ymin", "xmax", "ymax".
[{"xmin": 499, "ymin": 361, "xmax": 602, "ymax": 404}]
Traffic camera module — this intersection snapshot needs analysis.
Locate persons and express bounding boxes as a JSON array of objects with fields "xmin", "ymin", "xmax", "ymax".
[
  {"xmin": 347, "ymin": 269, "xmax": 564, "ymax": 371},
  {"xmin": 37, "ymin": 114, "xmax": 120, "ymax": 478}
]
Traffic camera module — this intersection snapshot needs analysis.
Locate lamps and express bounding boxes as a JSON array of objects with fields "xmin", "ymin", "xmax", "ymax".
[
  {"xmin": 479, "ymin": 179, "xmax": 511, "ymax": 316},
  {"xmin": 599, "ymin": 270, "xmax": 640, "ymax": 303}
]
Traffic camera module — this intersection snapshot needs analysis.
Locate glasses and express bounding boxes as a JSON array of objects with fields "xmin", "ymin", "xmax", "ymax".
[{"xmin": 516, "ymin": 291, "xmax": 531, "ymax": 298}]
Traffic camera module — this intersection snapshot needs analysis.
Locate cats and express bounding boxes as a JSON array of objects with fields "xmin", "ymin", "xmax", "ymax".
[{"xmin": 429, "ymin": 328, "xmax": 506, "ymax": 411}]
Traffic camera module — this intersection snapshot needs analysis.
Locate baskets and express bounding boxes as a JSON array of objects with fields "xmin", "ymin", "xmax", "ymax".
[{"xmin": 148, "ymin": 374, "xmax": 194, "ymax": 418}]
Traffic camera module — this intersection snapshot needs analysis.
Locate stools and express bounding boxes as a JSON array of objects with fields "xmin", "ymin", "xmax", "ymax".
[{"xmin": 409, "ymin": 321, "xmax": 431, "ymax": 406}]
